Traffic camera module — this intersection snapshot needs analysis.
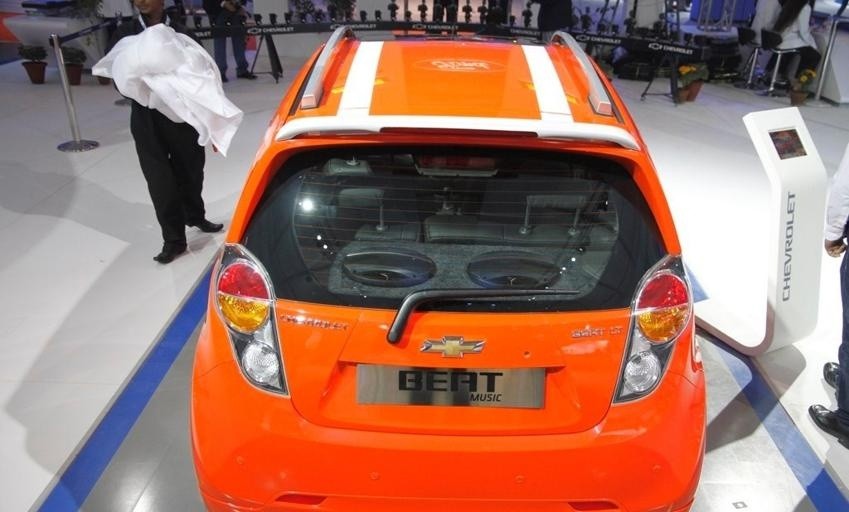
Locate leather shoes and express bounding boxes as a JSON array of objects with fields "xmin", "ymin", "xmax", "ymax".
[
  {"xmin": 809, "ymin": 403, "xmax": 849, "ymax": 448},
  {"xmin": 823, "ymin": 362, "xmax": 841, "ymax": 390}
]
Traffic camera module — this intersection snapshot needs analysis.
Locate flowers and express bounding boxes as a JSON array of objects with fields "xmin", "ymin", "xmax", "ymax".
[
  {"xmin": 797, "ymin": 69, "xmax": 817, "ymax": 93},
  {"xmin": 678, "ymin": 62, "xmax": 696, "ymax": 89}
]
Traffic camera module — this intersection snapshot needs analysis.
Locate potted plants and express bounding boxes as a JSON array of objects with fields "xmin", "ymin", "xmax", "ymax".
[
  {"xmin": 61, "ymin": 47, "xmax": 86, "ymax": 85},
  {"xmin": 686, "ymin": 63, "xmax": 709, "ymax": 102},
  {"xmin": 18, "ymin": 45, "xmax": 48, "ymax": 84}
]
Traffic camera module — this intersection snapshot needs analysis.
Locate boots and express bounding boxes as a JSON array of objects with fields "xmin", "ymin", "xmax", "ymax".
[{"xmin": 236, "ymin": 68, "xmax": 254, "ymax": 79}]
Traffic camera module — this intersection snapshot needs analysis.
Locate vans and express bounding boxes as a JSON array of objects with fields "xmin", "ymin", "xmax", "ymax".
[{"xmin": 189, "ymin": 26, "xmax": 705, "ymax": 511}]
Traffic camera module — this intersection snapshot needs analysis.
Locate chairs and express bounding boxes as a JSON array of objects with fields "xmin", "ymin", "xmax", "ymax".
[
  {"xmin": 332, "ymin": 170, "xmax": 454, "ymax": 242},
  {"xmin": 477, "ymin": 175, "xmax": 596, "ymax": 243}
]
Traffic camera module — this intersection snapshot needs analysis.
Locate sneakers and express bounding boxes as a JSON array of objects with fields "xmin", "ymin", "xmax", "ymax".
[
  {"xmin": 194, "ymin": 219, "xmax": 224, "ymax": 232},
  {"xmin": 158, "ymin": 241, "xmax": 186, "ymax": 263}
]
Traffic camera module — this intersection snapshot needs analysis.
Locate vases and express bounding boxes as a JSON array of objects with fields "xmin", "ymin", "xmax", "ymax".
[
  {"xmin": 790, "ymin": 90, "xmax": 809, "ymax": 107},
  {"xmin": 678, "ymin": 89, "xmax": 691, "ymax": 103}
]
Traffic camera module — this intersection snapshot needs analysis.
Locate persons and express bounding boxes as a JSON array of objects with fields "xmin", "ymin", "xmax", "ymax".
[
  {"xmin": 767, "ymin": 0, "xmax": 821, "ymax": 90},
  {"xmin": 114, "ymin": 0, "xmax": 225, "ymax": 264},
  {"xmin": 533, "ymin": 0, "xmax": 575, "ymax": 42},
  {"xmin": 203, "ymin": 1, "xmax": 258, "ymax": 83},
  {"xmin": 809, "ymin": 140, "xmax": 849, "ymax": 446}
]
